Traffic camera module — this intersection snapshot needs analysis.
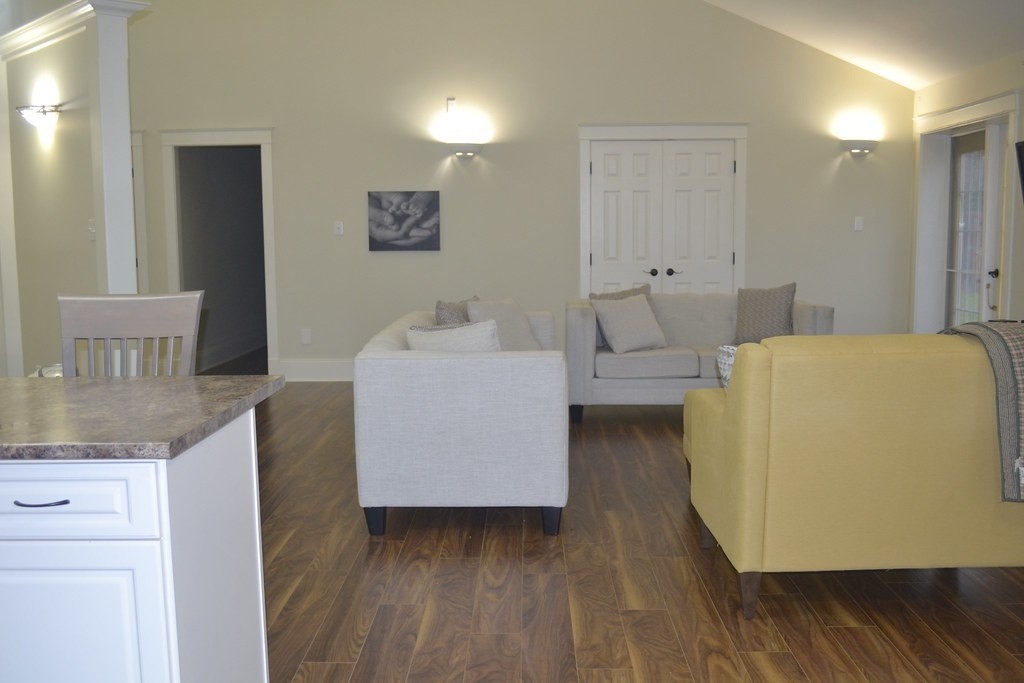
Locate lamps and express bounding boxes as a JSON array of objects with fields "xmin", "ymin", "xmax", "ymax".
[
  {"xmin": 447, "ymin": 142, "xmax": 484, "ymax": 160},
  {"xmin": 15, "ymin": 103, "xmax": 62, "ymax": 128},
  {"xmin": 841, "ymin": 140, "xmax": 879, "ymax": 158}
]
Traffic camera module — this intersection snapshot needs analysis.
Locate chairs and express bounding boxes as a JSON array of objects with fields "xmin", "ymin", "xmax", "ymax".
[{"xmin": 56, "ymin": 290, "xmax": 206, "ymax": 377}]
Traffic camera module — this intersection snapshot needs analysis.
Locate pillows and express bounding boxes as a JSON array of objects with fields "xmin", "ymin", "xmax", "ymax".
[
  {"xmin": 466, "ymin": 297, "xmax": 542, "ymax": 351},
  {"xmin": 590, "ymin": 293, "xmax": 667, "ymax": 354},
  {"xmin": 733, "ymin": 281, "xmax": 796, "ymax": 345},
  {"xmin": 435, "ymin": 295, "xmax": 480, "ymax": 325},
  {"xmin": 410, "ymin": 321, "xmax": 477, "ymax": 332},
  {"xmin": 406, "ymin": 319, "xmax": 501, "ymax": 353},
  {"xmin": 589, "ymin": 282, "xmax": 660, "ymax": 350},
  {"xmin": 716, "ymin": 343, "xmax": 737, "ymax": 395}
]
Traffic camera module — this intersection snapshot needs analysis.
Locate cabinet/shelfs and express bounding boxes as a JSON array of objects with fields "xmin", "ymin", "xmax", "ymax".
[{"xmin": 0, "ymin": 375, "xmax": 285, "ymax": 683}]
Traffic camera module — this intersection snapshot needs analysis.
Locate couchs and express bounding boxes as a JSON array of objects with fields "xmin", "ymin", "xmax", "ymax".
[
  {"xmin": 683, "ymin": 334, "xmax": 1024, "ymax": 620},
  {"xmin": 354, "ymin": 309, "xmax": 570, "ymax": 536},
  {"xmin": 564, "ymin": 292, "xmax": 835, "ymax": 426}
]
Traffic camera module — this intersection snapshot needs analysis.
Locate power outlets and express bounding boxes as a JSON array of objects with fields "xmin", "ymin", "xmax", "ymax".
[{"xmin": 301, "ymin": 328, "xmax": 312, "ymax": 345}]
